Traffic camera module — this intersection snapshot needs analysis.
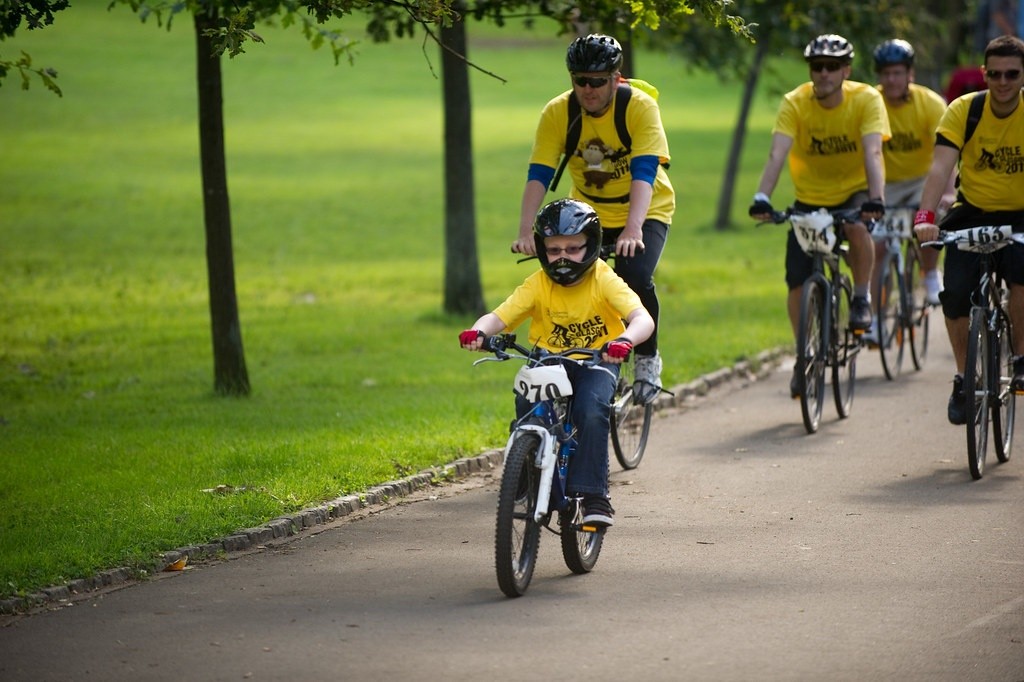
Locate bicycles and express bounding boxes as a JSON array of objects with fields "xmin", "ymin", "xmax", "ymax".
[
  {"xmin": 921, "ymin": 229, "xmax": 1024, "ymax": 479},
  {"xmin": 874, "ymin": 203, "xmax": 937, "ymax": 381},
  {"xmin": 511, "ymin": 243, "xmax": 652, "ymax": 470},
  {"xmin": 471, "ymin": 332, "xmax": 612, "ymax": 599},
  {"xmin": 756, "ymin": 209, "xmax": 865, "ymax": 434}
]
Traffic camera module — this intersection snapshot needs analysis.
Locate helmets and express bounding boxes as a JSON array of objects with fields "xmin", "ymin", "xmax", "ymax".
[
  {"xmin": 872, "ymin": 38, "xmax": 914, "ymax": 72},
  {"xmin": 803, "ymin": 33, "xmax": 855, "ymax": 67},
  {"xmin": 566, "ymin": 32, "xmax": 623, "ymax": 72},
  {"xmin": 532, "ymin": 197, "xmax": 603, "ymax": 288}
]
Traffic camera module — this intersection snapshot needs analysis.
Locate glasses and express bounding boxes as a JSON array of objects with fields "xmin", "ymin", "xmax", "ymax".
[
  {"xmin": 569, "ymin": 71, "xmax": 614, "ymax": 89},
  {"xmin": 986, "ymin": 69, "xmax": 1020, "ymax": 81},
  {"xmin": 546, "ymin": 243, "xmax": 587, "ymax": 255},
  {"xmin": 809, "ymin": 60, "xmax": 842, "ymax": 73}
]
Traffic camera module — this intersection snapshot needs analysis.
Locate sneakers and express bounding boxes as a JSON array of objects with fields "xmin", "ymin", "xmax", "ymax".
[
  {"xmin": 583, "ymin": 495, "xmax": 615, "ymax": 527},
  {"xmin": 633, "ymin": 349, "xmax": 663, "ymax": 404}
]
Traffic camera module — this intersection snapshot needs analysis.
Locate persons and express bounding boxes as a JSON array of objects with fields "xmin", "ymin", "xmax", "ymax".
[
  {"xmin": 458, "ymin": 198, "xmax": 656, "ymax": 527},
  {"xmin": 865, "ymin": 39, "xmax": 958, "ymax": 350},
  {"xmin": 746, "ymin": 34, "xmax": 892, "ymax": 402},
  {"xmin": 510, "ymin": 34, "xmax": 676, "ymax": 409},
  {"xmin": 913, "ymin": 36, "xmax": 1024, "ymax": 425}
]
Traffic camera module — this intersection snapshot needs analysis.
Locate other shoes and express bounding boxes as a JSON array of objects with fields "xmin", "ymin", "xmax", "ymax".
[
  {"xmin": 848, "ymin": 295, "xmax": 873, "ymax": 331},
  {"xmin": 947, "ymin": 373, "xmax": 983, "ymax": 424},
  {"xmin": 923, "ymin": 276, "xmax": 945, "ymax": 305},
  {"xmin": 790, "ymin": 363, "xmax": 802, "ymax": 395},
  {"xmin": 859, "ymin": 315, "xmax": 888, "ymax": 344},
  {"xmin": 1009, "ymin": 356, "xmax": 1024, "ymax": 395}
]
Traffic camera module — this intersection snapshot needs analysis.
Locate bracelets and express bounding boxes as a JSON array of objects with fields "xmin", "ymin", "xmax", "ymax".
[
  {"xmin": 914, "ymin": 210, "xmax": 935, "ymax": 224},
  {"xmin": 754, "ymin": 193, "xmax": 770, "ymax": 202}
]
